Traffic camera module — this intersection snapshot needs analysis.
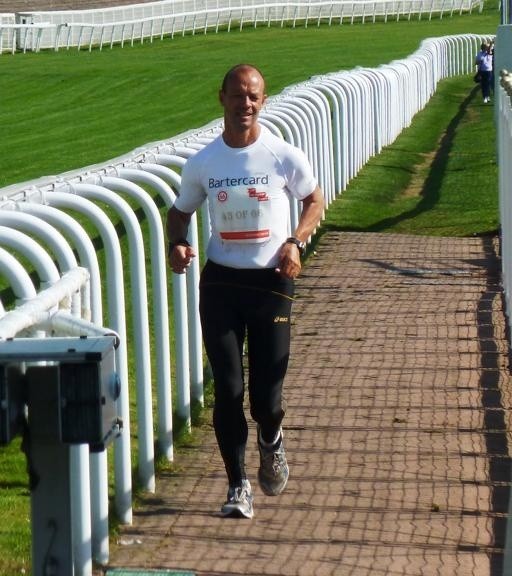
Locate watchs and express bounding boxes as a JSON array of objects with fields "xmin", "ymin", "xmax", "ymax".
[{"xmin": 286, "ymin": 237, "xmax": 305, "ymax": 257}]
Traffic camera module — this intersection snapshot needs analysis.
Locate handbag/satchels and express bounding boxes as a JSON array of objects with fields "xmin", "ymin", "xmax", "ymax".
[{"xmin": 474, "ymin": 72, "xmax": 482, "ymax": 83}]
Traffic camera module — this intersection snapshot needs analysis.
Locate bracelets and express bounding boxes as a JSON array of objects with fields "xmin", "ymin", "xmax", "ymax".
[{"xmin": 167, "ymin": 238, "xmax": 191, "ymax": 253}]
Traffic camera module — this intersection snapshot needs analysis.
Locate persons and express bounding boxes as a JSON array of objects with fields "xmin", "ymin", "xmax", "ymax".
[
  {"xmin": 166, "ymin": 63, "xmax": 325, "ymax": 521},
  {"xmin": 488, "ymin": 40, "xmax": 494, "ymax": 92},
  {"xmin": 474, "ymin": 41, "xmax": 492, "ymax": 104}
]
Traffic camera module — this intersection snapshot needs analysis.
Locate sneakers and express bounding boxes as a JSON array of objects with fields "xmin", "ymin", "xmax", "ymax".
[
  {"xmin": 484, "ymin": 96, "xmax": 490, "ymax": 104},
  {"xmin": 221, "ymin": 478, "xmax": 254, "ymax": 519},
  {"xmin": 256, "ymin": 423, "xmax": 289, "ymax": 496}
]
